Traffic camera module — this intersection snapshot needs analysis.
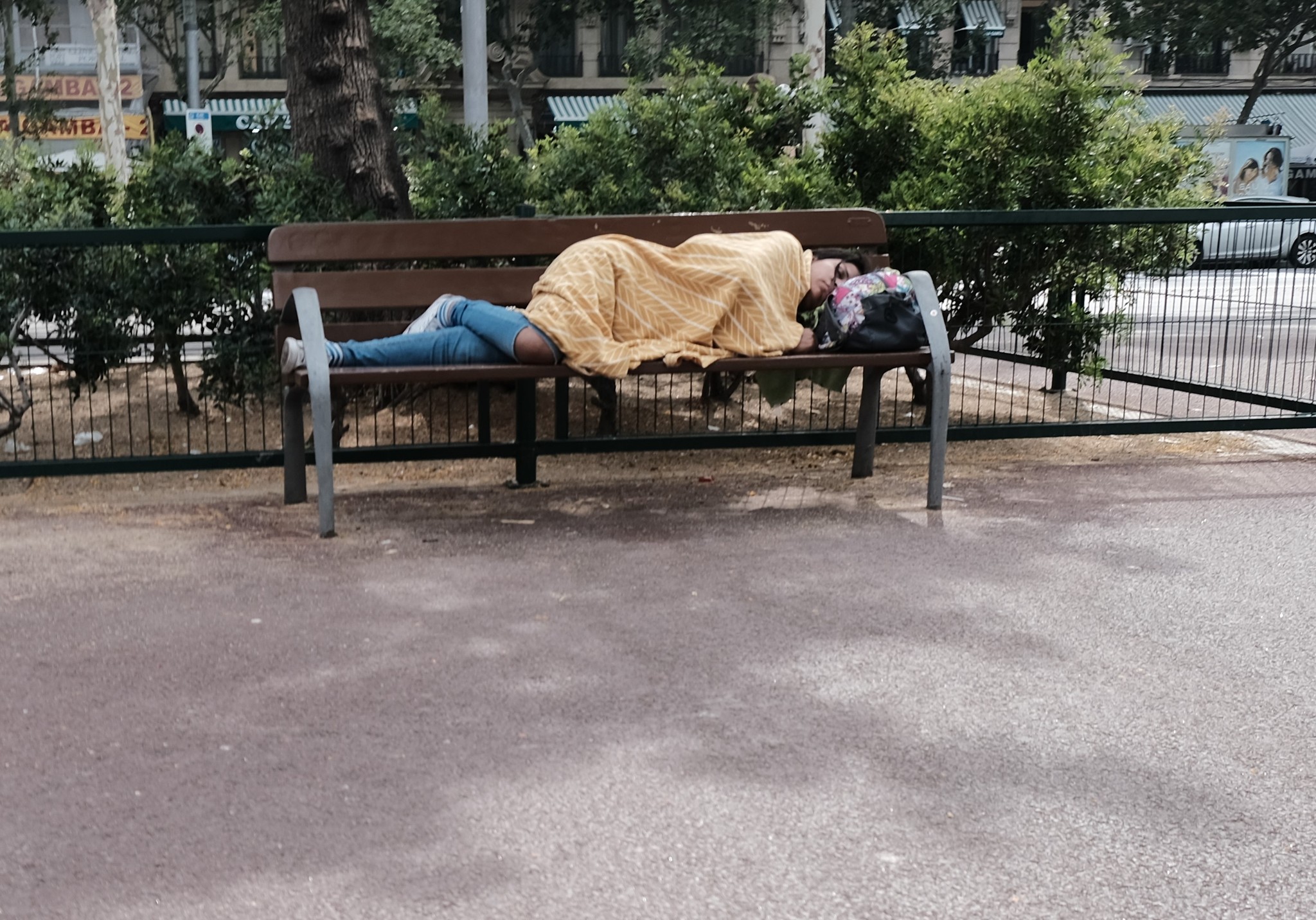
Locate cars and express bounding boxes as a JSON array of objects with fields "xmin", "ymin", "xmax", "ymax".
[{"xmin": 1150, "ymin": 196, "xmax": 1316, "ymax": 272}]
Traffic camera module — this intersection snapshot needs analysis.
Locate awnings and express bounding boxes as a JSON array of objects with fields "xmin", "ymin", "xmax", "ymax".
[
  {"xmin": 897, "ymin": 0, "xmax": 938, "ymax": 36},
  {"xmin": 825, "ymin": 0, "xmax": 875, "ymax": 31},
  {"xmin": 1086, "ymin": 94, "xmax": 1316, "ymax": 178},
  {"xmin": 547, "ymin": 95, "xmax": 638, "ymax": 134},
  {"xmin": 959, "ymin": 1, "xmax": 1007, "ymax": 38},
  {"xmin": 161, "ymin": 97, "xmax": 419, "ymax": 132}
]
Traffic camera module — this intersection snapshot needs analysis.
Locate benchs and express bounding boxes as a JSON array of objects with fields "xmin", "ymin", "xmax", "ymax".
[{"xmin": 265, "ymin": 205, "xmax": 957, "ymax": 542}]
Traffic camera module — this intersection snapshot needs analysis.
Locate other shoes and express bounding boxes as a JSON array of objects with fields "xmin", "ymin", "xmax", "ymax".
[
  {"xmin": 282, "ymin": 337, "xmax": 305, "ymax": 374},
  {"xmin": 402, "ymin": 293, "xmax": 460, "ymax": 334}
]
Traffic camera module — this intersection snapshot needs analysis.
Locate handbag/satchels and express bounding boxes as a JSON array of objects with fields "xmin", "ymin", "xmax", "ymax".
[{"xmin": 813, "ymin": 265, "xmax": 927, "ymax": 352}]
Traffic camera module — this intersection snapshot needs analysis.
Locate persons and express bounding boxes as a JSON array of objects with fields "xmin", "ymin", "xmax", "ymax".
[
  {"xmin": 279, "ymin": 230, "xmax": 869, "ymax": 377},
  {"xmin": 1234, "ymin": 147, "xmax": 1283, "ymax": 197}
]
